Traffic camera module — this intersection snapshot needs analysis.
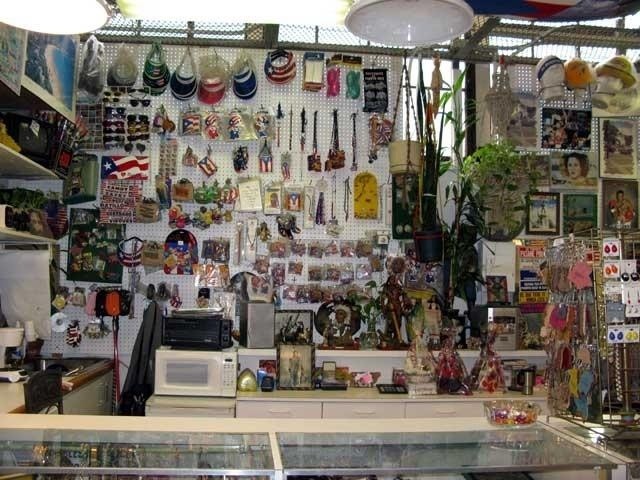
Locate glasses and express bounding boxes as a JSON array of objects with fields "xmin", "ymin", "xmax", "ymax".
[
  {"xmin": 111, "ymin": 87, "xmax": 125, "ymax": 92},
  {"xmin": 102, "ymin": 107, "xmax": 126, "ymax": 150},
  {"xmin": 129, "ymin": 100, "xmax": 150, "ymax": 107},
  {"xmin": 124, "ymin": 115, "xmax": 150, "ymax": 154},
  {"xmin": 128, "ymin": 89, "xmax": 146, "ymax": 99},
  {"xmin": 104, "ymin": 92, "xmax": 121, "ymax": 96},
  {"xmin": 102, "ymin": 98, "xmax": 119, "ymax": 102}
]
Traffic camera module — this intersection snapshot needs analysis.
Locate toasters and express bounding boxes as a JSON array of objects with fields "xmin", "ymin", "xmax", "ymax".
[{"xmin": 161, "ymin": 314, "xmax": 234, "ymax": 352}]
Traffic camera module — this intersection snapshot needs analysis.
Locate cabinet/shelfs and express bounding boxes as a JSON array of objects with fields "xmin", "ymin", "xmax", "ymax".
[{"xmin": 236, "ymin": 401, "xmax": 483, "ymax": 420}]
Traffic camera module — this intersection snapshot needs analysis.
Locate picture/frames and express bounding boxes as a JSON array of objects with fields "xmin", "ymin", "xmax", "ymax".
[
  {"xmin": 490, "ymin": 92, "xmax": 639, "ymax": 236},
  {"xmin": 277, "ymin": 341, "xmax": 315, "ymax": 390},
  {"xmin": 275, "ymin": 309, "xmax": 314, "ymax": 341}
]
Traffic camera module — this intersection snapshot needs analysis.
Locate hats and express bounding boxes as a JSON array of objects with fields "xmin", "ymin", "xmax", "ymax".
[
  {"xmin": 107, "ymin": 47, "xmax": 138, "ymax": 85},
  {"xmin": 143, "ymin": 42, "xmax": 170, "ymax": 95},
  {"xmin": 264, "ymin": 48, "xmax": 296, "ymax": 84},
  {"xmin": 117, "ymin": 236, "xmax": 144, "ymax": 268},
  {"xmin": 595, "ymin": 57, "xmax": 636, "ymax": 88},
  {"xmin": 197, "ymin": 56, "xmax": 227, "ymax": 104},
  {"xmin": 171, "ymin": 48, "xmax": 198, "ymax": 101},
  {"xmin": 537, "ymin": 57, "xmax": 567, "ymax": 81}
]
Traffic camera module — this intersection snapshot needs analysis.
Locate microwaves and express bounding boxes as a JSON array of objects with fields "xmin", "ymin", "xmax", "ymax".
[{"xmin": 155, "ymin": 346, "xmax": 238, "ymax": 399}]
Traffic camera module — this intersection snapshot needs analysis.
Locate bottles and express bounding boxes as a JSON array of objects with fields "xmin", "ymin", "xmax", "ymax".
[{"xmin": 521, "ymin": 368, "xmax": 534, "ymax": 395}]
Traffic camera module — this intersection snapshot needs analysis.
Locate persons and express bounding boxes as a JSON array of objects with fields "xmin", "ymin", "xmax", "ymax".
[
  {"xmin": 610, "ymin": 189, "xmax": 636, "ymax": 223},
  {"xmin": 563, "ymin": 153, "xmax": 585, "ymax": 184}
]
